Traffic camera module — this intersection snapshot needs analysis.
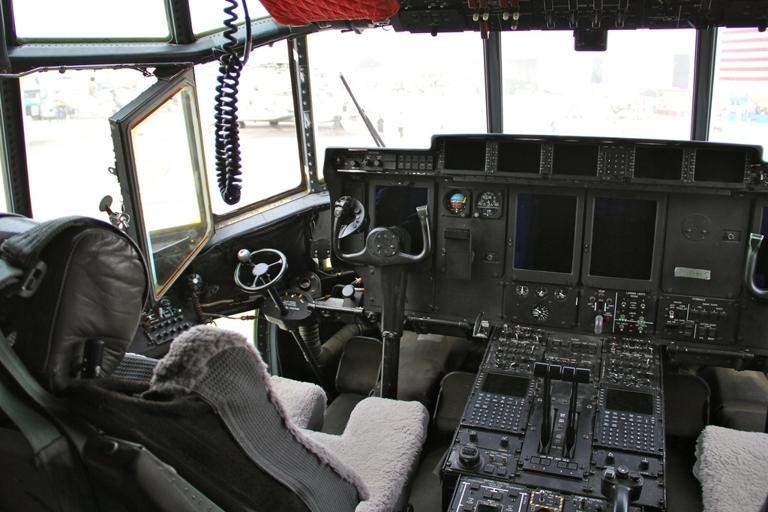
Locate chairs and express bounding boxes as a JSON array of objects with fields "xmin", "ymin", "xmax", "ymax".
[{"xmin": 0, "ymin": 211, "xmax": 429, "ymax": 510}]
